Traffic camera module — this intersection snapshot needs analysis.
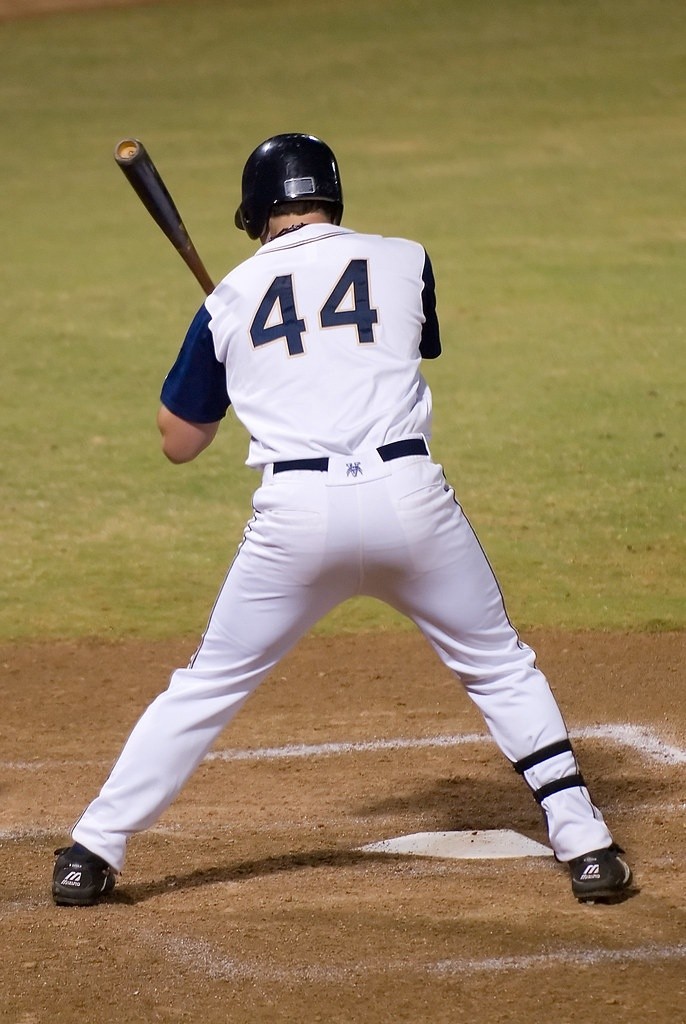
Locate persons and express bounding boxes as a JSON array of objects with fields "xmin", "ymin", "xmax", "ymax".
[{"xmin": 52, "ymin": 133, "xmax": 633, "ymax": 905}]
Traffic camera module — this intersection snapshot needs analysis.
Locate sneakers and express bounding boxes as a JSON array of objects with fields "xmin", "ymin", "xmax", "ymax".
[
  {"xmin": 568, "ymin": 843, "xmax": 633, "ymax": 905},
  {"xmin": 51, "ymin": 846, "xmax": 116, "ymax": 905}
]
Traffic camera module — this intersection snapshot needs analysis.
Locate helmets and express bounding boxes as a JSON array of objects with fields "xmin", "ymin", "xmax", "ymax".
[{"xmin": 234, "ymin": 132, "xmax": 344, "ymax": 240}]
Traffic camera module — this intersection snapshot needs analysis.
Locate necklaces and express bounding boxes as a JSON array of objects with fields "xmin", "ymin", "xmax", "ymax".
[{"xmin": 269, "ymin": 222, "xmax": 306, "ymax": 240}]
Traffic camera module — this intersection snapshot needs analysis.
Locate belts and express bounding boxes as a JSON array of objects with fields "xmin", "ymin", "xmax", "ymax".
[{"xmin": 272, "ymin": 438, "xmax": 428, "ymax": 475}]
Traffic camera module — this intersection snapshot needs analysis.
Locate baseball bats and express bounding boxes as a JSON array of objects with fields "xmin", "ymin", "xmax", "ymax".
[{"xmin": 113, "ymin": 139, "xmax": 216, "ymax": 297}]
